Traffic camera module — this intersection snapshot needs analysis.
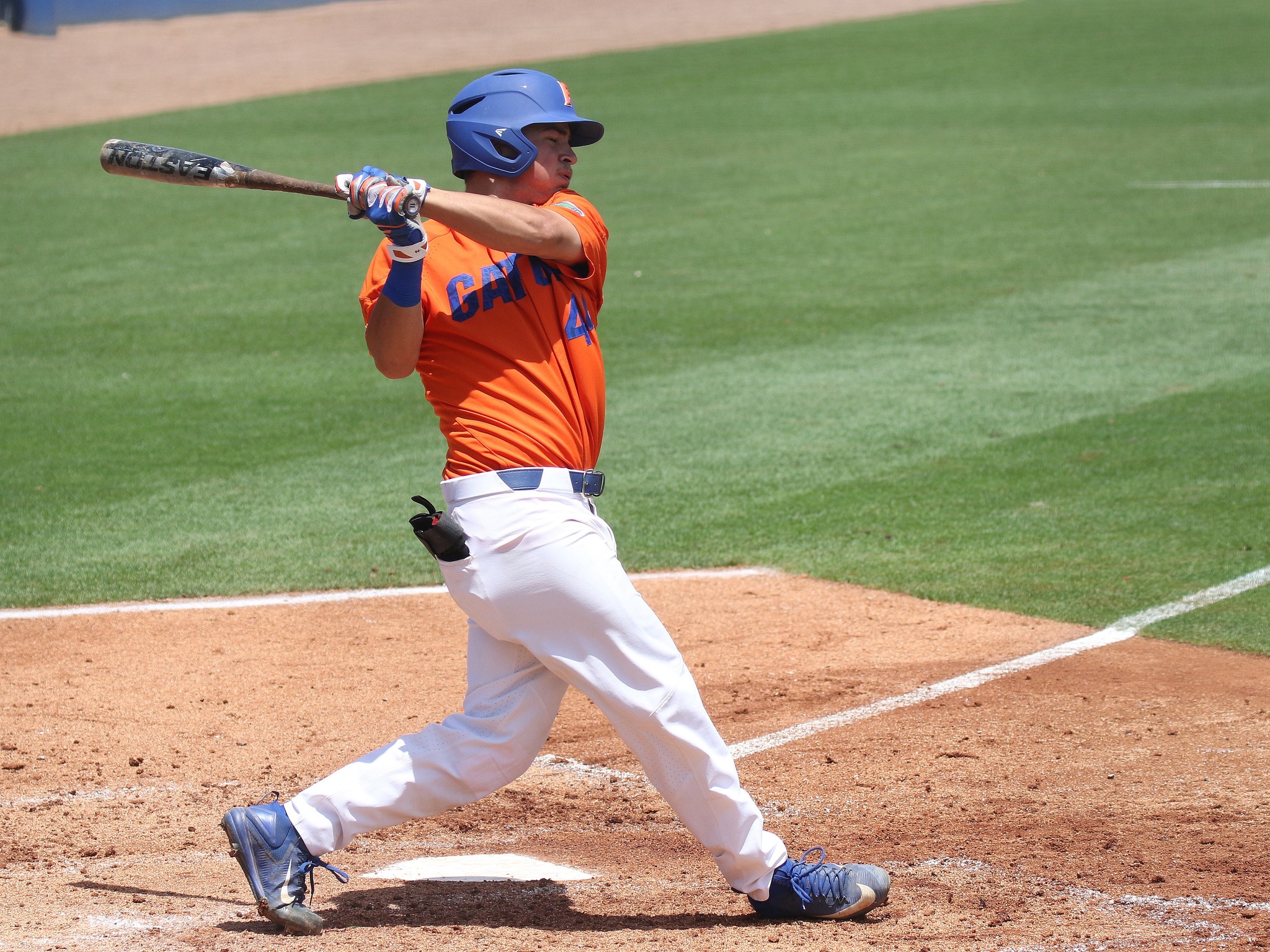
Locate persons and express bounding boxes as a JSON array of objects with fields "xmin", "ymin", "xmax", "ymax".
[{"xmin": 220, "ymin": 67, "xmax": 891, "ymax": 938}]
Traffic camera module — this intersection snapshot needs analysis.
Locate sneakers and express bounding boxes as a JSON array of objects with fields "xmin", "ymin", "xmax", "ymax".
[
  {"xmin": 748, "ymin": 847, "xmax": 890, "ymax": 920},
  {"xmin": 218, "ymin": 790, "xmax": 350, "ymax": 934}
]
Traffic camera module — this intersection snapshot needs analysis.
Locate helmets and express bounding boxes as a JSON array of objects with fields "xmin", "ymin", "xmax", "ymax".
[{"xmin": 447, "ymin": 68, "xmax": 604, "ymax": 179}]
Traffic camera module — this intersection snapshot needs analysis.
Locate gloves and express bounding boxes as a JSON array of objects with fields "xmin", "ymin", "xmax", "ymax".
[{"xmin": 334, "ymin": 165, "xmax": 431, "ymax": 263}]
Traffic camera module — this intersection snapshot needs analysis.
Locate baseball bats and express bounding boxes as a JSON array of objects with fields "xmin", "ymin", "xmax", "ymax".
[{"xmin": 99, "ymin": 138, "xmax": 421, "ymax": 218}]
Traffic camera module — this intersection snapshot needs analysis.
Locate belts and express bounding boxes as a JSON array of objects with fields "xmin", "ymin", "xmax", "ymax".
[{"xmin": 497, "ymin": 469, "xmax": 606, "ymax": 497}]
